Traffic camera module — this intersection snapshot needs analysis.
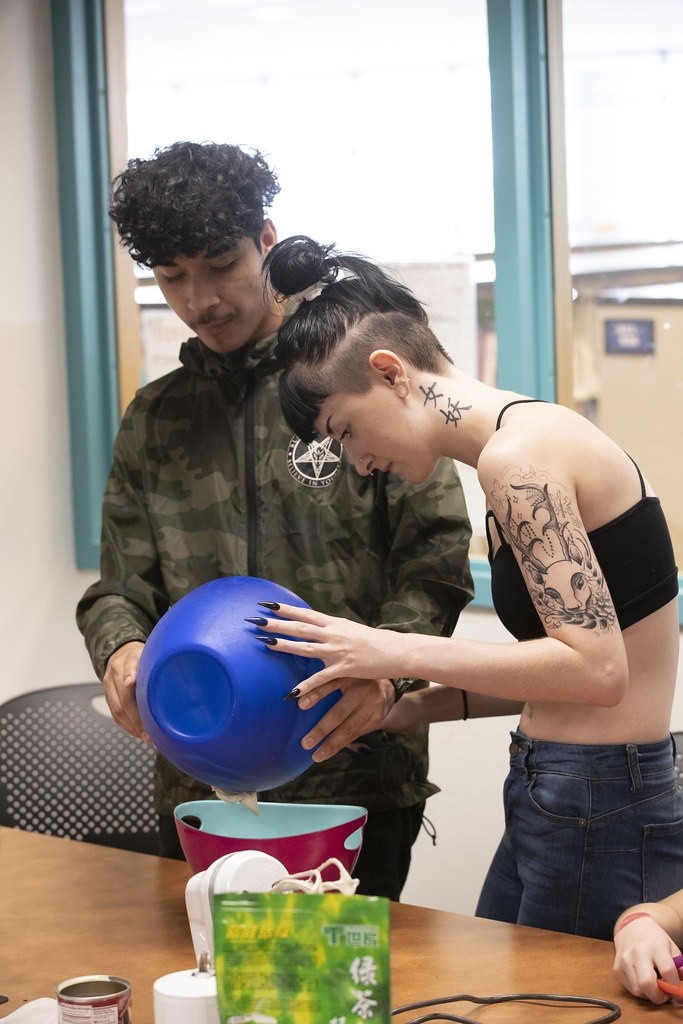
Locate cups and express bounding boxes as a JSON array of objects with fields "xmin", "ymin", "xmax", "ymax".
[{"xmin": 153, "ymin": 970, "xmax": 220, "ymax": 1024}]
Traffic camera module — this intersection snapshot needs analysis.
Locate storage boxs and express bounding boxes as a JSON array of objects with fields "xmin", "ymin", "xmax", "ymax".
[{"xmin": 210, "ymin": 891, "xmax": 395, "ymax": 1024}]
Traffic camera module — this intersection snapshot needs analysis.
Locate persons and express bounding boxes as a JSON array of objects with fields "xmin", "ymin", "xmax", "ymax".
[
  {"xmin": 74, "ymin": 138, "xmax": 478, "ymax": 904},
  {"xmin": 614, "ymin": 888, "xmax": 683, "ymax": 1006},
  {"xmin": 243, "ymin": 233, "xmax": 683, "ymax": 942}
]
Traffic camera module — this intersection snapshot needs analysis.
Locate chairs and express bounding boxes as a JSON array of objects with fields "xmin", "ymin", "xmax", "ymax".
[{"xmin": 0, "ymin": 683, "xmax": 192, "ymax": 863}]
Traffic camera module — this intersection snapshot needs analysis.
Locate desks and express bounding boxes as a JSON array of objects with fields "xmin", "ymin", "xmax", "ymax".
[{"xmin": 0, "ymin": 825, "xmax": 683, "ymax": 1024}]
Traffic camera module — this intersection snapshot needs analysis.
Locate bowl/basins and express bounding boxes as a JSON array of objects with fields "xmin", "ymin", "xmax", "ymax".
[
  {"xmin": 135, "ymin": 576, "xmax": 334, "ymax": 793},
  {"xmin": 173, "ymin": 800, "xmax": 373, "ymax": 895}
]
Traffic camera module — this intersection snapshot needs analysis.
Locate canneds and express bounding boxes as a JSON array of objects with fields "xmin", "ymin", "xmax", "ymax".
[{"xmin": 56, "ymin": 974, "xmax": 131, "ymax": 1024}]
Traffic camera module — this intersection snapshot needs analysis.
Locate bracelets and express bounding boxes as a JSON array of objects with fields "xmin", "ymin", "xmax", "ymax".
[{"xmin": 461, "ymin": 690, "xmax": 470, "ymax": 721}]
